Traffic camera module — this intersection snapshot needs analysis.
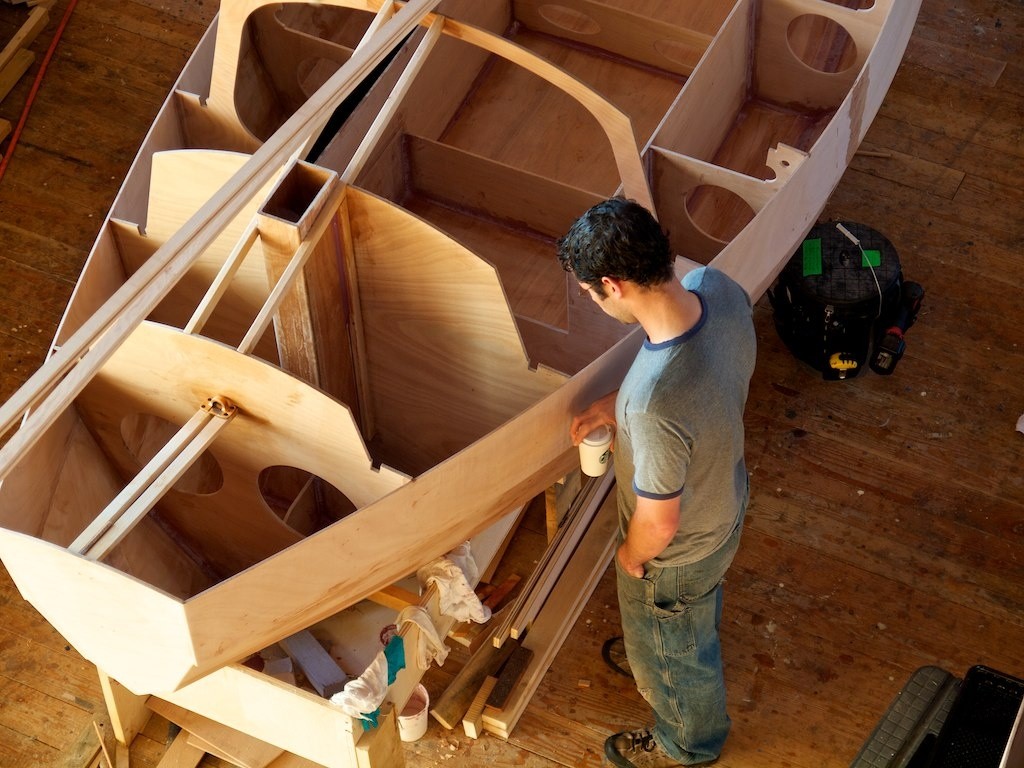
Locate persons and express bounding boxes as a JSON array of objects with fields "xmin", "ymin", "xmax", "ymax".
[{"xmin": 554, "ymin": 199, "xmax": 756, "ymax": 768}]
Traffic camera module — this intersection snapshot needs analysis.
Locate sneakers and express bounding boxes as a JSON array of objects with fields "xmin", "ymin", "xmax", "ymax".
[
  {"xmin": 604, "ymin": 725, "xmax": 720, "ymax": 768},
  {"xmin": 602, "ymin": 636, "xmax": 635, "ymax": 679}
]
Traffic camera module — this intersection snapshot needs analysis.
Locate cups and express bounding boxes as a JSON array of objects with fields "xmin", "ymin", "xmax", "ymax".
[{"xmin": 579, "ymin": 424, "xmax": 613, "ymax": 477}]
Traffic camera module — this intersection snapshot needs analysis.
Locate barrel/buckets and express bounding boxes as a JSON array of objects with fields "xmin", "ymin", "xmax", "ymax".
[{"xmin": 397, "ymin": 683, "xmax": 430, "ymax": 742}]
